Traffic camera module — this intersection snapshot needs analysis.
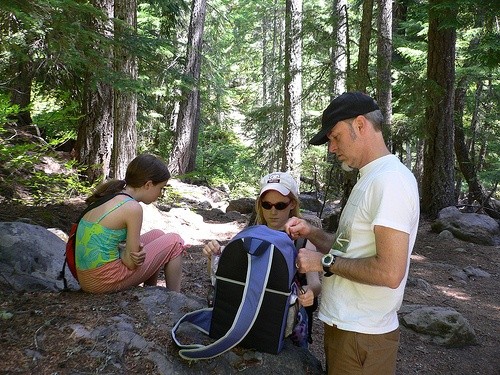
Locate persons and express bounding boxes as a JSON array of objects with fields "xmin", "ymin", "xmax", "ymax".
[
  {"xmin": 203, "ymin": 171, "xmax": 321, "ymax": 353},
  {"xmin": 283, "ymin": 91, "xmax": 420, "ymax": 375},
  {"xmin": 74, "ymin": 154, "xmax": 185, "ymax": 294}
]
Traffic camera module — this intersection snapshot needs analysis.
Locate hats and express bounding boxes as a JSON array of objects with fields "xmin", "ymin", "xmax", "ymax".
[
  {"xmin": 258, "ymin": 172, "xmax": 298, "ymax": 198},
  {"xmin": 308, "ymin": 91, "xmax": 381, "ymax": 146}
]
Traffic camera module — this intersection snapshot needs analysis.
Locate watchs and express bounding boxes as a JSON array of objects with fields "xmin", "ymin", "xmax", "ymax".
[{"xmin": 321, "ymin": 253, "xmax": 337, "ymax": 277}]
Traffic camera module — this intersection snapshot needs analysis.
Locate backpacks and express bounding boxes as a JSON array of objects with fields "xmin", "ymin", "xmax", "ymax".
[{"xmin": 170, "ymin": 224, "xmax": 300, "ymax": 360}]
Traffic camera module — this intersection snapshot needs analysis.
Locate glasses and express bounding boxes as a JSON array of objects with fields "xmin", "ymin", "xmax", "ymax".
[{"xmin": 261, "ymin": 200, "xmax": 293, "ymax": 209}]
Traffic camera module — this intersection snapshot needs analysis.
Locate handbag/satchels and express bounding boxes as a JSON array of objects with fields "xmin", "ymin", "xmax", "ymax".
[{"xmin": 66, "ymin": 223, "xmax": 77, "ymax": 280}]
